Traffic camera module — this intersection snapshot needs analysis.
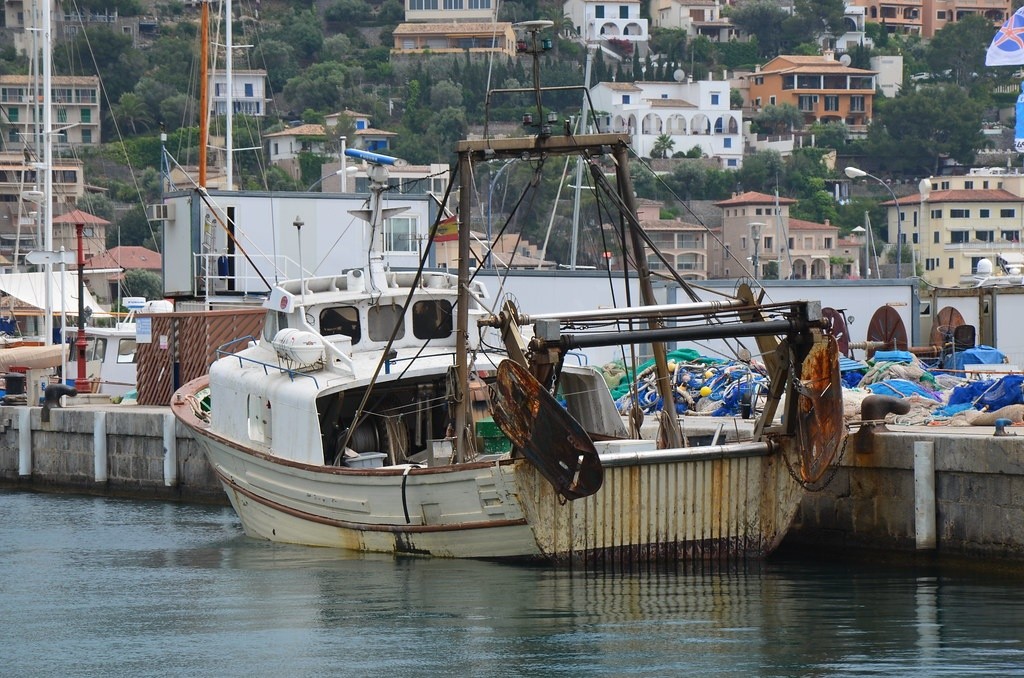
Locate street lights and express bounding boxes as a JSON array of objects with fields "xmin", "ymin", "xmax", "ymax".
[{"xmin": 844, "ymin": 166, "xmax": 902, "ymax": 279}]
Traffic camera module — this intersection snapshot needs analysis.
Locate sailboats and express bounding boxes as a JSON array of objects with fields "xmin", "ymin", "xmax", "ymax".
[{"xmin": 1, "ymin": 1, "xmax": 154, "ymax": 405}]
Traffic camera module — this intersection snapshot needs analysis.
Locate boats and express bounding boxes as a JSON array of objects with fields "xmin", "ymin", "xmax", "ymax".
[{"xmin": 164, "ymin": 1, "xmax": 851, "ymax": 569}]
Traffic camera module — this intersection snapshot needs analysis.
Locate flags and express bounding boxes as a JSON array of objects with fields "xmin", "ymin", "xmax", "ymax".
[{"xmin": 428, "ymin": 215, "xmax": 459, "ymax": 243}]
